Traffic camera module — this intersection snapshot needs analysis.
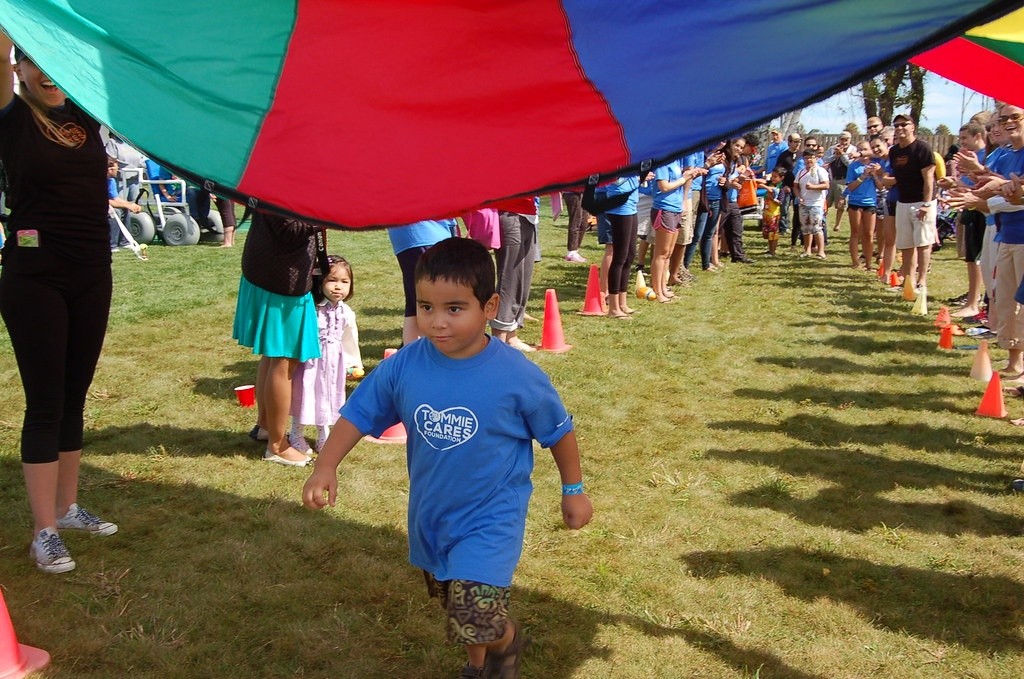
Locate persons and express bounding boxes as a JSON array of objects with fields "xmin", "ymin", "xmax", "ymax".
[
  {"xmin": 105, "ymin": 129, "xmax": 236, "ymax": 252},
  {"xmin": 0, "ymin": 31, "xmax": 118, "ymax": 572},
  {"xmin": 300, "ymin": 238, "xmax": 593, "ymax": 679},
  {"xmin": 232, "ymin": 99, "xmax": 1024, "ymax": 466}
]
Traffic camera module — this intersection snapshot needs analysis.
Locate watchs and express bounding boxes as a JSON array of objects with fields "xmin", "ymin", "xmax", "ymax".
[{"xmin": 921, "ymin": 203, "xmax": 931, "ymax": 207}]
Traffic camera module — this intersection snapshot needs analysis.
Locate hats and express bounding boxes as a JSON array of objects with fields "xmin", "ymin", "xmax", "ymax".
[
  {"xmin": 839, "ymin": 131, "xmax": 852, "ymax": 138},
  {"xmin": 892, "ymin": 114, "xmax": 915, "ymax": 124},
  {"xmin": 788, "ymin": 133, "xmax": 803, "ymax": 142},
  {"xmin": 771, "ymin": 128, "xmax": 782, "ymax": 134}
]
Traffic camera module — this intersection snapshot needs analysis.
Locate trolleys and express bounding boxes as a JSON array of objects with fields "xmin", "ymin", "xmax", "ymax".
[{"xmin": 122, "ymin": 168, "xmax": 224, "ymax": 247}]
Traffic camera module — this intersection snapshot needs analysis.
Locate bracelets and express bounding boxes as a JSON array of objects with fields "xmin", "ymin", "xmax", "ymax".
[
  {"xmin": 861, "ymin": 174, "xmax": 866, "ymax": 178},
  {"xmin": 873, "ymin": 176, "xmax": 878, "ymax": 179},
  {"xmin": 561, "ymin": 481, "xmax": 583, "ymax": 496},
  {"xmin": 857, "ymin": 177, "xmax": 863, "ymax": 183}
]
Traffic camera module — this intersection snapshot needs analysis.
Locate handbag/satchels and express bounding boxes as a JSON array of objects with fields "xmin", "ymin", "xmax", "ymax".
[{"xmin": 738, "ymin": 179, "xmax": 760, "ymax": 215}]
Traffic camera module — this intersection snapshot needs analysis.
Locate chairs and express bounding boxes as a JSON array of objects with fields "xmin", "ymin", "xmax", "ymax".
[{"xmin": 145, "ymin": 159, "xmax": 190, "ymax": 206}]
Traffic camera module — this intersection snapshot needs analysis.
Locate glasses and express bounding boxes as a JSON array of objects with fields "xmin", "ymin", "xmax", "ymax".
[
  {"xmin": 893, "ymin": 123, "xmax": 912, "ymax": 128},
  {"xmin": 984, "ymin": 124, "xmax": 991, "ymax": 132},
  {"xmin": 805, "ymin": 144, "xmax": 817, "ymax": 147},
  {"xmin": 866, "ymin": 124, "xmax": 880, "ymax": 129},
  {"xmin": 999, "ymin": 114, "xmax": 1024, "ymax": 125}
]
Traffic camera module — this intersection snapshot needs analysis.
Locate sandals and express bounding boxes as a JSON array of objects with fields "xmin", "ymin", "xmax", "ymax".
[{"xmin": 459, "ymin": 625, "xmax": 532, "ymax": 679}]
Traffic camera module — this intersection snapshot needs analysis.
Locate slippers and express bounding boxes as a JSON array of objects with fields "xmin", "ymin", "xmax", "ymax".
[
  {"xmin": 666, "ymin": 281, "xmax": 691, "ymax": 288},
  {"xmin": 628, "ymin": 310, "xmax": 641, "ymax": 316},
  {"xmin": 801, "ymin": 252, "xmax": 827, "ymax": 261},
  {"xmin": 660, "ymin": 295, "xmax": 680, "ymax": 304},
  {"xmin": 606, "ymin": 316, "xmax": 633, "ymax": 320}
]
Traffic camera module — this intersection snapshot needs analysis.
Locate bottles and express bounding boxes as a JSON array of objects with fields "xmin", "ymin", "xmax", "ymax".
[{"xmin": 910, "ymin": 206, "xmax": 929, "ymax": 224}]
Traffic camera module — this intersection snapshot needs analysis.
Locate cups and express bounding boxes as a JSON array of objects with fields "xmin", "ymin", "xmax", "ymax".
[{"xmin": 233, "ymin": 384, "xmax": 256, "ymax": 407}]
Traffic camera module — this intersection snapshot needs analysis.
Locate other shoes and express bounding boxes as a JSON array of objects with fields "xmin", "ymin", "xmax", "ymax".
[
  {"xmin": 265, "ymin": 448, "xmax": 316, "ymax": 468},
  {"xmin": 250, "ymin": 425, "xmax": 269, "ymax": 441},
  {"xmin": 565, "ymin": 255, "xmax": 587, "ymax": 263},
  {"xmin": 948, "ymin": 291, "xmax": 983, "ymax": 307},
  {"xmin": 731, "ymin": 257, "xmax": 755, "ymax": 263}
]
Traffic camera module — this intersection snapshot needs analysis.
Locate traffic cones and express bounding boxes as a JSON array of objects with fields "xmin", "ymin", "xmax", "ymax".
[
  {"xmin": 974, "ymin": 370, "xmax": 1008, "ymax": 418},
  {"xmin": 969, "ymin": 340, "xmax": 993, "ymax": 380},
  {"xmin": 576, "ymin": 264, "xmax": 609, "ymax": 316},
  {"xmin": 909, "ymin": 286, "xmax": 929, "ymax": 316},
  {"xmin": 364, "ymin": 348, "xmax": 407, "ymax": 445},
  {"xmin": 537, "ymin": 289, "xmax": 573, "ymax": 354},
  {"xmin": 877, "ymin": 260, "xmax": 901, "ymax": 287},
  {"xmin": 937, "ymin": 324, "xmax": 953, "ymax": 350},
  {"xmin": 933, "ymin": 307, "xmax": 951, "ymax": 327},
  {"xmin": 902, "ymin": 275, "xmax": 917, "ymax": 301}
]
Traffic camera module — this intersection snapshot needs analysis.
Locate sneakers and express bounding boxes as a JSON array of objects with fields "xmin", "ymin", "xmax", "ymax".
[
  {"xmin": 30, "ymin": 527, "xmax": 76, "ymax": 574},
  {"xmin": 56, "ymin": 503, "xmax": 119, "ymax": 537}
]
